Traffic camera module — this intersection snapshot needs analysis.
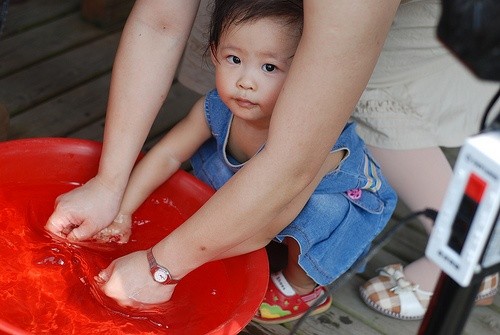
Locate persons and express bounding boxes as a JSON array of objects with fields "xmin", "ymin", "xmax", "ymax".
[
  {"xmin": 92, "ymin": 0, "xmax": 399, "ymax": 324},
  {"xmin": 44, "ymin": 0, "xmax": 500, "ymax": 320}
]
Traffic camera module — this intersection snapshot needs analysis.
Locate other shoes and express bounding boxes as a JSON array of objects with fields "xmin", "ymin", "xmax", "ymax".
[
  {"xmin": 359, "ymin": 263, "xmax": 499, "ymax": 321},
  {"xmin": 250, "ymin": 272, "xmax": 332, "ymax": 324}
]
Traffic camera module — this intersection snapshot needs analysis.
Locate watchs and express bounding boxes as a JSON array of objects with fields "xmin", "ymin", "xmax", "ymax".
[{"xmin": 147, "ymin": 247, "xmax": 180, "ymax": 285}]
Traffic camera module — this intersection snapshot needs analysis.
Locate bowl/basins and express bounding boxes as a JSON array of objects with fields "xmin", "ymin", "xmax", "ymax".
[{"xmin": 0, "ymin": 137, "xmax": 269, "ymax": 335}]
